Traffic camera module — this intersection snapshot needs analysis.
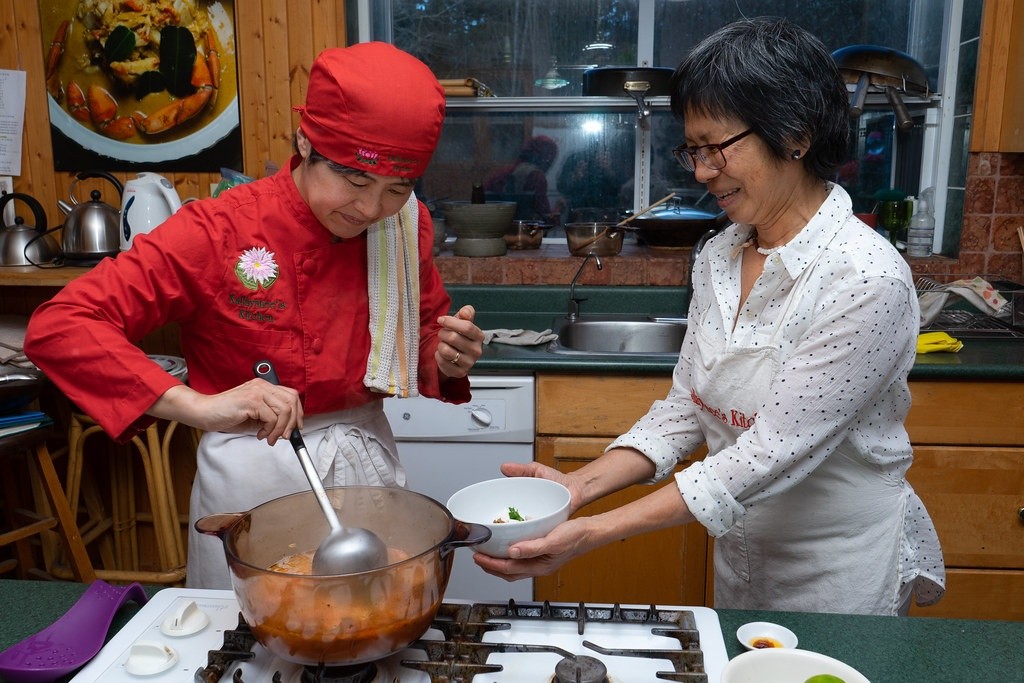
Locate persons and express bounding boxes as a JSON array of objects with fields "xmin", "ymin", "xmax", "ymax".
[
  {"xmin": 473, "ymin": 15, "xmax": 945, "ymax": 616},
  {"xmin": 558, "ymin": 125, "xmax": 623, "ymax": 207},
  {"xmin": 609, "ymin": 126, "xmax": 667, "ymax": 211},
  {"xmin": 481, "ymin": 133, "xmax": 566, "ymax": 230},
  {"xmin": 22, "ymin": 42, "xmax": 485, "ymax": 591}
]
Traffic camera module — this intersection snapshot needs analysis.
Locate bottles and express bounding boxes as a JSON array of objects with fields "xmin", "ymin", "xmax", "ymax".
[{"xmin": 907, "ymin": 200, "xmax": 935, "ymax": 257}]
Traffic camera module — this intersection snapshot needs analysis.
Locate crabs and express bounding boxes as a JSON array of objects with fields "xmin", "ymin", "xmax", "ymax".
[{"xmin": 47, "ymin": 0, "xmax": 221, "ymax": 138}]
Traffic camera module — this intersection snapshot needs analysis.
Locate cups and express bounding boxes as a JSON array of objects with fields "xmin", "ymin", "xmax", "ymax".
[
  {"xmin": 854, "ymin": 212, "xmax": 879, "ymax": 231},
  {"xmin": 882, "ymin": 200, "xmax": 913, "ymax": 253}
]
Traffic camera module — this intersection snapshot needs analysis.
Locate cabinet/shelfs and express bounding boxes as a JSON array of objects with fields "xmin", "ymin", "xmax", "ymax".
[
  {"xmin": 969, "ymin": 0, "xmax": 1024, "ymax": 156},
  {"xmin": 534, "ymin": 373, "xmax": 1024, "ymax": 621}
]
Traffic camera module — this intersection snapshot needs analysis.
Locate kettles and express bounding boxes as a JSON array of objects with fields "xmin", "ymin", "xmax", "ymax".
[
  {"xmin": 55, "ymin": 169, "xmax": 125, "ymax": 267},
  {"xmin": 0, "ymin": 192, "xmax": 63, "ymax": 267},
  {"xmin": 119, "ymin": 173, "xmax": 183, "ymax": 252}
]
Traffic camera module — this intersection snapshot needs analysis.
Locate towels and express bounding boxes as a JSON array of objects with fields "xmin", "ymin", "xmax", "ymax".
[{"xmin": 361, "ymin": 189, "xmax": 423, "ymax": 399}]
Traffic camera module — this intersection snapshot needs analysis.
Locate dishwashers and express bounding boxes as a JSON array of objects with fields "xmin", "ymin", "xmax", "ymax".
[{"xmin": 384, "ymin": 375, "xmax": 535, "ymax": 602}]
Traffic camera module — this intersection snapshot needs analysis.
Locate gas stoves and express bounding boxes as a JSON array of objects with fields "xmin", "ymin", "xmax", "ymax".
[{"xmin": 66, "ymin": 587, "xmax": 730, "ymax": 683}]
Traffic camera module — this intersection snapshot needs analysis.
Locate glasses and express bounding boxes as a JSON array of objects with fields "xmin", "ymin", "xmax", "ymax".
[{"xmin": 672, "ymin": 126, "xmax": 757, "ymax": 171}]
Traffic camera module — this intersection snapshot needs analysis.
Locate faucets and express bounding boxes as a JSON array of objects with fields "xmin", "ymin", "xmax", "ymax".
[{"xmin": 567, "ymin": 252, "xmax": 603, "ymax": 320}]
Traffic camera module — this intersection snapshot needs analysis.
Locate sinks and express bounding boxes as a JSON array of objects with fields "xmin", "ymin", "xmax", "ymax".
[{"xmin": 558, "ymin": 321, "xmax": 689, "ymax": 354}]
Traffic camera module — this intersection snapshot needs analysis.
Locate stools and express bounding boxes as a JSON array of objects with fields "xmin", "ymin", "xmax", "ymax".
[{"xmin": 0, "ymin": 354, "xmax": 202, "ymax": 589}]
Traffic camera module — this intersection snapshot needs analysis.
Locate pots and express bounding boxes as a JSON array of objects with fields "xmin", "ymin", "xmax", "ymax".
[
  {"xmin": 580, "ymin": 66, "xmax": 675, "ymax": 120},
  {"xmin": 503, "ymin": 219, "xmax": 554, "ymax": 250},
  {"xmin": 194, "ymin": 483, "xmax": 493, "ymax": 668},
  {"xmin": 831, "ymin": 43, "xmax": 928, "ymax": 129},
  {"xmin": 620, "ymin": 196, "xmax": 730, "ymax": 247},
  {"xmin": 564, "ymin": 221, "xmax": 641, "ymax": 258}
]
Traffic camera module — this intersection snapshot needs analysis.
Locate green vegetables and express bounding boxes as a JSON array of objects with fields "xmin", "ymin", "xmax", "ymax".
[
  {"xmin": 104, "ymin": 25, "xmax": 198, "ymax": 101},
  {"xmin": 508, "ymin": 507, "xmax": 525, "ymax": 521}
]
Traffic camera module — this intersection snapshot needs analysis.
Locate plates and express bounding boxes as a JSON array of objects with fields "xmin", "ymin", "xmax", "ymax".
[{"xmin": 38, "ymin": 0, "xmax": 239, "ymax": 163}]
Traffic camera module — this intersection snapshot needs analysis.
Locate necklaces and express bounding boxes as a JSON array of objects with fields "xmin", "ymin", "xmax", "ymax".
[{"xmin": 731, "ymin": 231, "xmax": 784, "ymax": 260}]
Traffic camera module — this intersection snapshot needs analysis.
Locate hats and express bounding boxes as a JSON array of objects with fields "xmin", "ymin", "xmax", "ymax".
[{"xmin": 292, "ymin": 41, "xmax": 447, "ymax": 178}]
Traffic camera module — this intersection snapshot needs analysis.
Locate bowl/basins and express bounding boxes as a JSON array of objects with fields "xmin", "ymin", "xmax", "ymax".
[
  {"xmin": 446, "ymin": 477, "xmax": 571, "ymax": 560},
  {"xmin": 719, "ymin": 647, "xmax": 870, "ymax": 683},
  {"xmin": 736, "ymin": 621, "xmax": 798, "ymax": 650}
]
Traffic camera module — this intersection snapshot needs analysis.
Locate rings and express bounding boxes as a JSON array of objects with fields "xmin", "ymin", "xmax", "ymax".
[{"xmin": 450, "ymin": 351, "xmax": 460, "ymax": 364}]
